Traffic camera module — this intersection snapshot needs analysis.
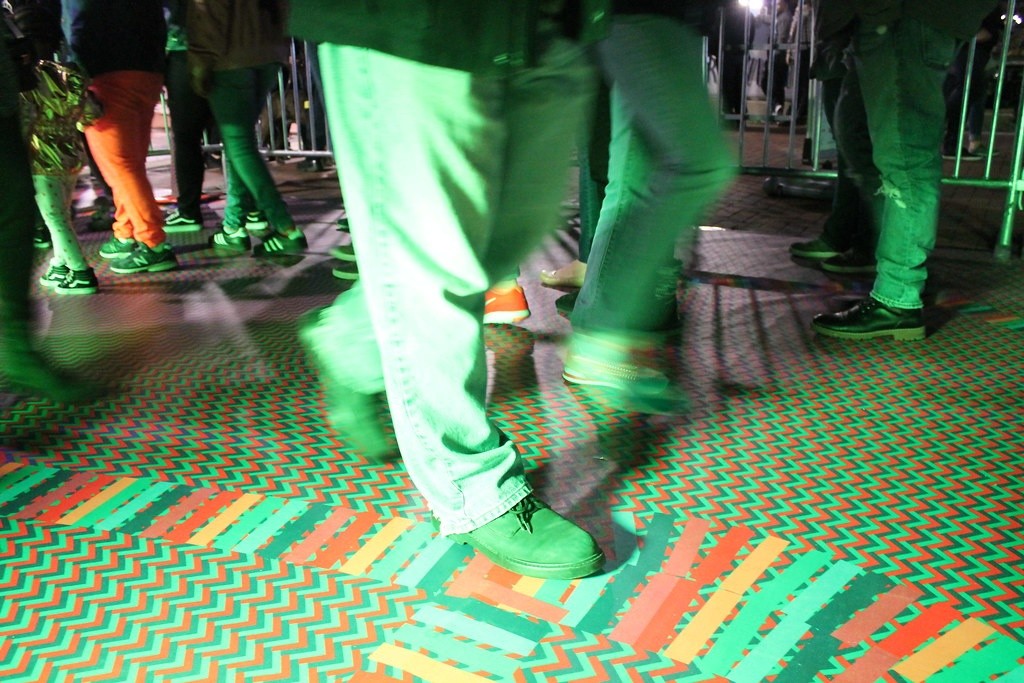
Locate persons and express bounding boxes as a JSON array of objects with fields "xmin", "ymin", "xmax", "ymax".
[
  {"xmin": 484, "ymin": 87, "xmax": 611, "ymax": 322},
  {"xmin": 561, "ymin": 0, "xmax": 738, "ymax": 395},
  {"xmin": 708, "ymin": 0, "xmax": 756, "ymax": 119},
  {"xmin": 759, "ymin": 0, "xmax": 1004, "ymax": 341},
  {"xmin": 0, "ymin": 0, "xmax": 331, "ymax": 403},
  {"xmin": 288, "ymin": 0, "xmax": 605, "ymax": 580}
]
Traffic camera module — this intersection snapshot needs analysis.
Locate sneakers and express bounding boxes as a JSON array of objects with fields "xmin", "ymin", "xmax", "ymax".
[
  {"xmin": 432, "ymin": 487, "xmax": 607, "ymax": 581},
  {"xmin": 161, "ymin": 208, "xmax": 203, "ymax": 232},
  {"xmin": 222, "ymin": 212, "xmax": 269, "ymax": 230},
  {"xmin": 99, "ymin": 236, "xmax": 139, "ymax": 258},
  {"xmin": 109, "ymin": 242, "xmax": 178, "ymax": 273},
  {"xmin": 296, "ymin": 305, "xmax": 399, "ymax": 459},
  {"xmin": 40, "ymin": 263, "xmax": 69, "ymax": 286},
  {"xmin": 55, "ymin": 267, "xmax": 99, "ymax": 295},
  {"xmin": 208, "ymin": 227, "xmax": 252, "ymax": 250},
  {"xmin": 252, "ymin": 230, "xmax": 309, "ymax": 253},
  {"xmin": 33, "ymin": 230, "xmax": 51, "ymax": 249}
]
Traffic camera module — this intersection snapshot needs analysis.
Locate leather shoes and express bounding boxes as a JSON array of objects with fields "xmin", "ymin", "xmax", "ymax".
[{"xmin": 811, "ymin": 296, "xmax": 927, "ymax": 341}]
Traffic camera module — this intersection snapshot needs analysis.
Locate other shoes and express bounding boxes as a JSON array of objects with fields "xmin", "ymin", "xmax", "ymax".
[
  {"xmin": 331, "ymin": 243, "xmax": 356, "ymax": 261},
  {"xmin": 88, "ymin": 219, "xmax": 116, "ymax": 230},
  {"xmin": 790, "ymin": 239, "xmax": 843, "ymax": 258},
  {"xmin": 822, "ymin": 248, "xmax": 880, "ymax": 273},
  {"xmin": 540, "ymin": 260, "xmax": 584, "ymax": 287},
  {"xmin": 563, "ymin": 352, "xmax": 689, "ymax": 413},
  {"xmin": 942, "ymin": 147, "xmax": 981, "ymax": 162},
  {"xmin": 554, "ymin": 288, "xmax": 681, "ymax": 332},
  {"xmin": 89, "ymin": 210, "xmax": 113, "ymax": 219},
  {"xmin": 483, "ymin": 285, "xmax": 531, "ymax": 324},
  {"xmin": 332, "ymin": 263, "xmax": 360, "ymax": 280},
  {"xmin": 968, "ymin": 144, "xmax": 1000, "ymax": 156},
  {"xmin": 0, "ymin": 316, "xmax": 98, "ymax": 406},
  {"xmin": 297, "ymin": 159, "xmax": 322, "ymax": 172}
]
